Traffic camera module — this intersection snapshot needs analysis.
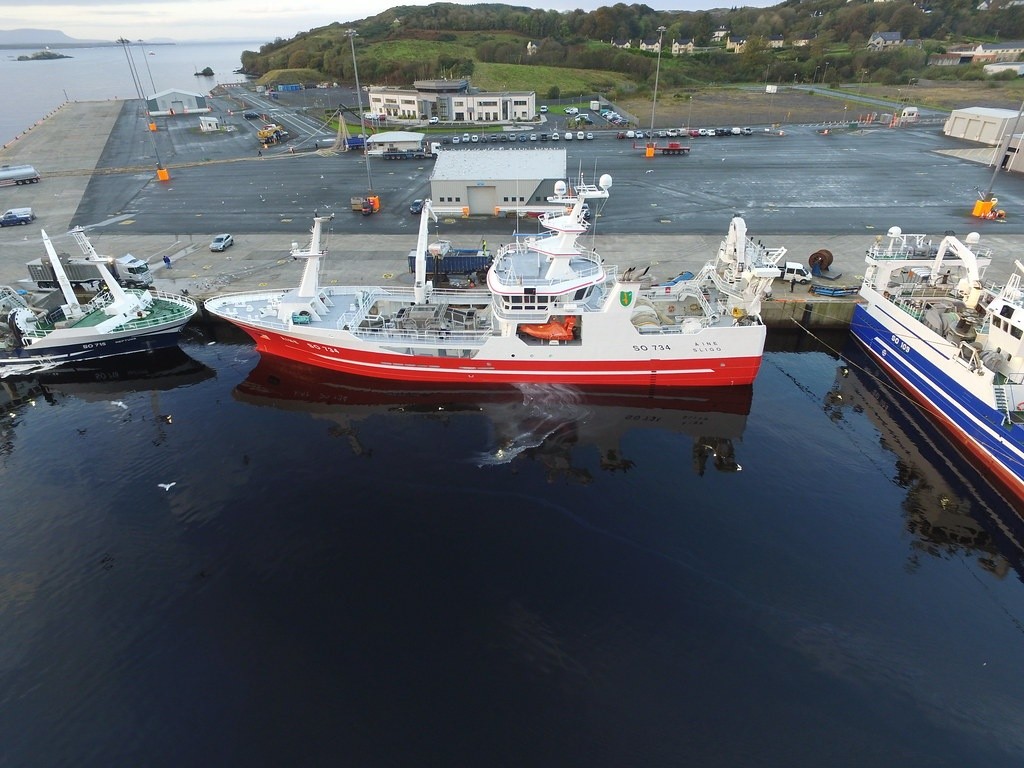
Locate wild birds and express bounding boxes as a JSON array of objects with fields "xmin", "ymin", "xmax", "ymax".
[
  {"xmin": 157, "ymin": 481, "xmax": 177, "ymax": 492},
  {"xmin": 111, "ymin": 401, "xmax": 129, "ymax": 410}
]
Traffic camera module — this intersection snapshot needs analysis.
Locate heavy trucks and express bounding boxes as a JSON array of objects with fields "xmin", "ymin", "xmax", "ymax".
[
  {"xmin": 0, "ymin": 165, "xmax": 41, "ymax": 186},
  {"xmin": 408, "ymin": 248, "xmax": 494, "ymax": 283},
  {"xmin": 26, "ymin": 253, "xmax": 153, "ymax": 290}
]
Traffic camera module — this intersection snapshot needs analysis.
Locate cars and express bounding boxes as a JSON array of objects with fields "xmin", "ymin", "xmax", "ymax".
[
  {"xmin": 410, "ymin": 199, "xmax": 424, "ymax": 214},
  {"xmin": 519, "ymin": 134, "xmax": 526, "ymax": 142},
  {"xmin": 689, "ymin": 127, "xmax": 753, "ymax": 137},
  {"xmin": 602, "ymin": 111, "xmax": 629, "ymax": 125},
  {"xmin": 564, "ymin": 107, "xmax": 593, "ymax": 125},
  {"xmin": 463, "ymin": 134, "xmax": 469, "ymax": 142},
  {"xmin": 541, "ymin": 134, "xmax": 547, "ymax": 141},
  {"xmin": 209, "ymin": 234, "xmax": 234, "ymax": 252},
  {"xmin": 491, "ymin": 134, "xmax": 497, "ymax": 142},
  {"xmin": 472, "ymin": 135, "xmax": 478, "ymax": 142},
  {"xmin": 540, "ymin": 106, "xmax": 548, "ymax": 113},
  {"xmin": 666, "ymin": 129, "xmax": 677, "ymax": 138},
  {"xmin": 529, "ymin": 134, "xmax": 537, "ymax": 140},
  {"xmin": 245, "ymin": 112, "xmax": 260, "ymax": 120},
  {"xmin": 0, "ymin": 214, "xmax": 32, "ymax": 228},
  {"xmin": 677, "ymin": 129, "xmax": 688, "ymax": 137},
  {"xmin": 581, "ymin": 203, "xmax": 590, "ymax": 219},
  {"xmin": 617, "ymin": 132, "xmax": 624, "ymax": 139},
  {"xmin": 657, "ymin": 131, "xmax": 667, "ymax": 138},
  {"xmin": 635, "ymin": 131, "xmax": 643, "ymax": 138},
  {"xmin": 552, "ymin": 133, "xmax": 559, "ymax": 140},
  {"xmin": 429, "ymin": 117, "xmax": 438, "ymax": 124},
  {"xmin": 564, "ymin": 133, "xmax": 573, "ymax": 140},
  {"xmin": 481, "ymin": 136, "xmax": 487, "ymax": 143},
  {"xmin": 500, "ymin": 134, "xmax": 507, "ymax": 142},
  {"xmin": 453, "ymin": 137, "xmax": 459, "ymax": 143},
  {"xmin": 587, "ymin": 133, "xmax": 593, "ymax": 140},
  {"xmin": 577, "ymin": 131, "xmax": 584, "ymax": 140},
  {"xmin": 626, "ymin": 131, "xmax": 635, "ymax": 139},
  {"xmin": 509, "ymin": 134, "xmax": 516, "ymax": 141},
  {"xmin": 644, "ymin": 131, "xmax": 654, "ymax": 138}
]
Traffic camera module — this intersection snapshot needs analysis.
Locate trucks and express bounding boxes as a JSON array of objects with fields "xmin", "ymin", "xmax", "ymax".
[
  {"xmin": 339, "ymin": 103, "xmax": 376, "ymax": 150},
  {"xmin": 778, "ymin": 261, "xmax": 812, "ymax": 285},
  {"xmin": 383, "ymin": 142, "xmax": 441, "ymax": 160}
]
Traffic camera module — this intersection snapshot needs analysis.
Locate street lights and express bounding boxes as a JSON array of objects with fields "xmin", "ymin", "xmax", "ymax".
[
  {"xmin": 324, "ymin": 94, "xmax": 333, "ymax": 122},
  {"xmin": 650, "ymin": 26, "xmax": 667, "ymax": 147},
  {"xmin": 811, "ymin": 62, "xmax": 919, "ymax": 109},
  {"xmin": 688, "ymin": 96, "xmax": 692, "ymax": 128},
  {"xmin": 116, "ymin": 37, "xmax": 163, "ymax": 170},
  {"xmin": 343, "ymin": 29, "xmax": 374, "ymax": 197}
]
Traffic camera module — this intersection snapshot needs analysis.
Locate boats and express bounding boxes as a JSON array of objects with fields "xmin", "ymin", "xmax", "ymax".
[
  {"xmin": 0, "ymin": 346, "xmax": 217, "ymax": 441},
  {"xmin": 849, "ymin": 226, "xmax": 1024, "ymax": 503},
  {"xmin": 230, "ymin": 350, "xmax": 754, "ymax": 486},
  {"xmin": 824, "ymin": 339, "xmax": 1023, "ymax": 585},
  {"xmin": 202, "ymin": 157, "xmax": 787, "ymax": 385},
  {"xmin": 0, "ymin": 224, "xmax": 199, "ymax": 381}
]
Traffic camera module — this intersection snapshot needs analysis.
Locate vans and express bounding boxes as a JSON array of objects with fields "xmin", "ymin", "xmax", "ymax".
[{"xmin": 0, "ymin": 208, "xmax": 36, "ymax": 221}]
